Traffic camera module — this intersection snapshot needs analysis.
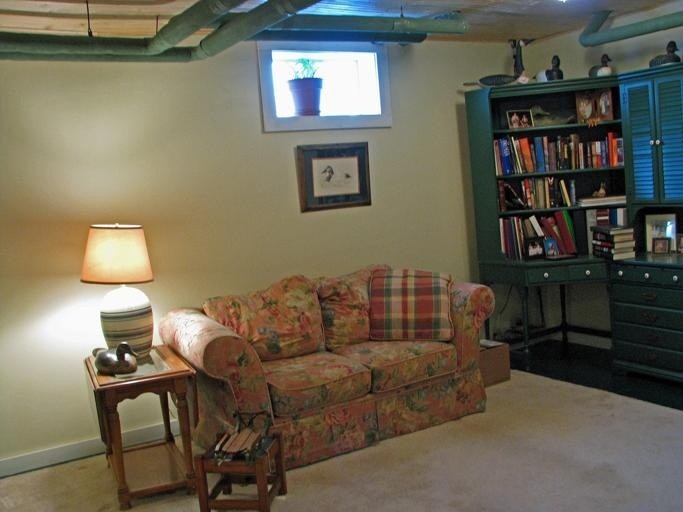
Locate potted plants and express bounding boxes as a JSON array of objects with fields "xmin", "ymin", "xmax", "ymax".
[{"xmin": 286, "ymin": 58, "xmax": 323, "ymax": 116}]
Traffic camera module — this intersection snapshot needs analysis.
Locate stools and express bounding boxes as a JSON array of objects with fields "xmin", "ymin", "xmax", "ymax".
[{"xmin": 194, "ymin": 430, "xmax": 287, "ymax": 512}]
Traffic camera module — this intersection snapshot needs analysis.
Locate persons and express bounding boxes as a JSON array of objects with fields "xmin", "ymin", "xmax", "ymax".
[
  {"xmin": 315, "ymin": 161, "xmax": 336, "ymax": 195},
  {"xmin": 511, "ymin": 111, "xmax": 529, "ymax": 128}
]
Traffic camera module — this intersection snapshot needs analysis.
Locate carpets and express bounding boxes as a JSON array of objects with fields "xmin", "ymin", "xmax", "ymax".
[{"xmin": 0, "ymin": 367, "xmax": 682, "ymax": 510}]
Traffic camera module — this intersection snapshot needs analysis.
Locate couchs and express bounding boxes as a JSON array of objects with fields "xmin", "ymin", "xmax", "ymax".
[{"xmin": 158, "ymin": 263, "xmax": 495, "ymax": 471}]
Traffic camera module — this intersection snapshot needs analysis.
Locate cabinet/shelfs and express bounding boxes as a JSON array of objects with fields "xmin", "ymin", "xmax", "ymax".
[
  {"xmin": 618, "ymin": 61, "xmax": 683, "ymax": 207},
  {"xmin": 464, "ymin": 74, "xmax": 631, "ymax": 372},
  {"xmin": 608, "ymin": 259, "xmax": 683, "ymax": 382}
]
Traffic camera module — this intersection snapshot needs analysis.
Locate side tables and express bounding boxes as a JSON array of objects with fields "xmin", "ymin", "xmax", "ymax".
[{"xmin": 84, "ymin": 344, "xmax": 200, "ymax": 512}]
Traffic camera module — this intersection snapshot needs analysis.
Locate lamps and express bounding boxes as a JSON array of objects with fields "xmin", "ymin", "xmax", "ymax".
[{"xmin": 80, "ymin": 222, "xmax": 154, "ymax": 360}]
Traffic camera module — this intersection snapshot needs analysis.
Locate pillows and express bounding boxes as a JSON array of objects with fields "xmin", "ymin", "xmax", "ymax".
[
  {"xmin": 369, "ymin": 262, "xmax": 455, "ymax": 342},
  {"xmin": 310, "ymin": 261, "xmax": 398, "ymax": 352},
  {"xmin": 201, "ymin": 274, "xmax": 326, "ymax": 361}
]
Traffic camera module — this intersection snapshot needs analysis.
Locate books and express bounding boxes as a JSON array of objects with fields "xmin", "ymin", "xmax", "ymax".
[{"xmin": 493, "ymin": 132, "xmax": 639, "ymax": 261}]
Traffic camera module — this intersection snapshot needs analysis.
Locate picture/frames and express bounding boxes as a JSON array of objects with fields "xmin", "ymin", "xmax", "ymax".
[
  {"xmin": 296, "ymin": 142, "xmax": 372, "ymax": 213},
  {"xmin": 525, "ymin": 236, "xmax": 546, "ymax": 261},
  {"xmin": 506, "ymin": 109, "xmax": 534, "ymax": 129},
  {"xmin": 645, "ymin": 213, "xmax": 677, "ymax": 253},
  {"xmin": 652, "ymin": 237, "xmax": 672, "ymax": 256}
]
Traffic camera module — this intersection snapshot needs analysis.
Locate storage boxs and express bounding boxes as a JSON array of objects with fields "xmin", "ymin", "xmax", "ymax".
[{"xmin": 478, "ymin": 339, "xmax": 511, "ymax": 387}]
